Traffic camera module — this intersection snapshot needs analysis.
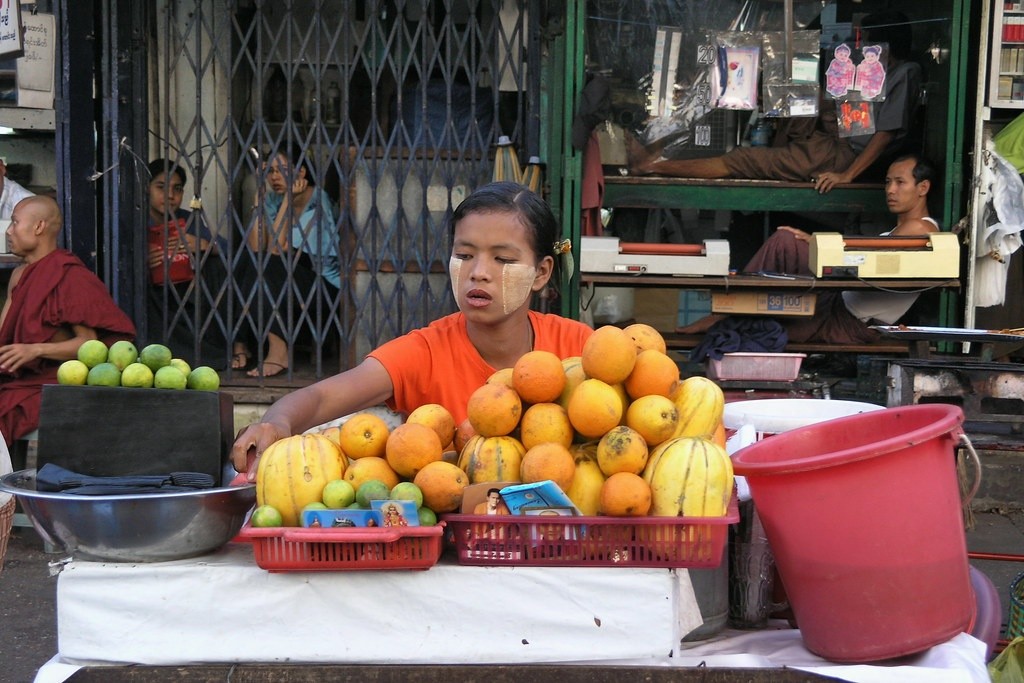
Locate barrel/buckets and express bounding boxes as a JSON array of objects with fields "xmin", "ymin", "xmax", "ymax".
[{"xmin": 730, "ymin": 404, "xmax": 981, "ymax": 665}]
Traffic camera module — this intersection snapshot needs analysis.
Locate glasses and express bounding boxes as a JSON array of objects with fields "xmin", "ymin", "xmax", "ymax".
[{"xmin": 266, "ymin": 166, "xmax": 290, "ymax": 176}]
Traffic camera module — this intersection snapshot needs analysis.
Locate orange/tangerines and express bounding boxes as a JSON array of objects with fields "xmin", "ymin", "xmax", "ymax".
[
  {"xmin": 250, "ymin": 479, "xmax": 437, "ymax": 528},
  {"xmin": 55, "ymin": 340, "xmax": 219, "ymax": 392},
  {"xmin": 337, "ymin": 322, "xmax": 681, "ymax": 515}
]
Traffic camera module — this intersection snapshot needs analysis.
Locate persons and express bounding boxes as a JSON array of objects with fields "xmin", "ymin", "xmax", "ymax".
[
  {"xmin": 232, "ymin": 145, "xmax": 342, "ymax": 377},
  {"xmin": 674, "ymin": 155, "xmax": 939, "ymax": 344},
  {"xmin": 147, "ymin": 158, "xmax": 227, "ymax": 370},
  {"xmin": 623, "ymin": 12, "xmax": 923, "ymax": 194},
  {"xmin": 229, "ymin": 181, "xmax": 595, "ymax": 484},
  {"xmin": 0, "ymin": 160, "xmax": 36, "ymax": 254},
  {"xmin": 473, "ymin": 488, "xmax": 511, "ymax": 539},
  {"xmin": 533, "ymin": 511, "xmax": 562, "ymax": 557},
  {"xmin": 0, "ymin": 195, "xmax": 136, "ymax": 448}
]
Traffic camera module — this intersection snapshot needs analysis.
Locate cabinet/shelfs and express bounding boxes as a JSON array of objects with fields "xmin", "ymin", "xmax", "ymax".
[{"xmin": 986, "ymin": 0, "xmax": 1024, "ymax": 109}]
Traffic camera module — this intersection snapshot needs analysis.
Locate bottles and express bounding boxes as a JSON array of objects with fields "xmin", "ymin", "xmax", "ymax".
[{"xmin": 751, "ymin": 113, "xmax": 769, "ymax": 147}]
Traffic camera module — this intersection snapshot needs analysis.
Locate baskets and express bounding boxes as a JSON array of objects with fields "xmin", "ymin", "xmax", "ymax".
[
  {"xmin": 1, "ymin": 494, "xmax": 18, "ymax": 573},
  {"xmin": 233, "ymin": 503, "xmax": 448, "ymax": 573},
  {"xmin": 438, "ymin": 475, "xmax": 741, "ymax": 570},
  {"xmin": 1004, "ymin": 570, "xmax": 1024, "ymax": 640}
]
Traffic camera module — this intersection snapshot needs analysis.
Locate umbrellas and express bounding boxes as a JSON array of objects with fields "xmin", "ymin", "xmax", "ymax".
[{"xmin": 492, "ymin": 136, "xmax": 552, "ymax": 315}]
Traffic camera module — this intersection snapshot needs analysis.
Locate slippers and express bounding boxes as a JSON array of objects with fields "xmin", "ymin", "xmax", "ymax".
[
  {"xmin": 231, "ymin": 351, "xmax": 251, "ymax": 371},
  {"xmin": 246, "ymin": 361, "xmax": 289, "ymax": 379}
]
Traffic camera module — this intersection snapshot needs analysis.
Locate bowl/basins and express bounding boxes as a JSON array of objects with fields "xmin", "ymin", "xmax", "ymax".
[
  {"xmin": 722, "ymin": 398, "xmax": 888, "ymax": 441},
  {"xmin": 0, "ymin": 468, "xmax": 257, "ymax": 564}
]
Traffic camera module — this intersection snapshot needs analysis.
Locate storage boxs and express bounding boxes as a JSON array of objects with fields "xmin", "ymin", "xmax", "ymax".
[
  {"xmin": 706, "ymin": 353, "xmax": 807, "ymax": 381},
  {"xmin": 711, "ymin": 287, "xmax": 817, "ymax": 316}
]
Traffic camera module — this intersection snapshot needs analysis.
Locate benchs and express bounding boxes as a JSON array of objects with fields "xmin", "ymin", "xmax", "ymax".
[
  {"xmin": 655, "ymin": 333, "xmax": 912, "ymax": 353},
  {"xmin": 600, "ymin": 173, "xmax": 893, "ymax": 242}
]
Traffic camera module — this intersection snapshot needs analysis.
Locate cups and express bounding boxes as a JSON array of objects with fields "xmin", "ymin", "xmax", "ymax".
[{"xmin": 727, "ymin": 536, "xmax": 790, "ymax": 632}]
